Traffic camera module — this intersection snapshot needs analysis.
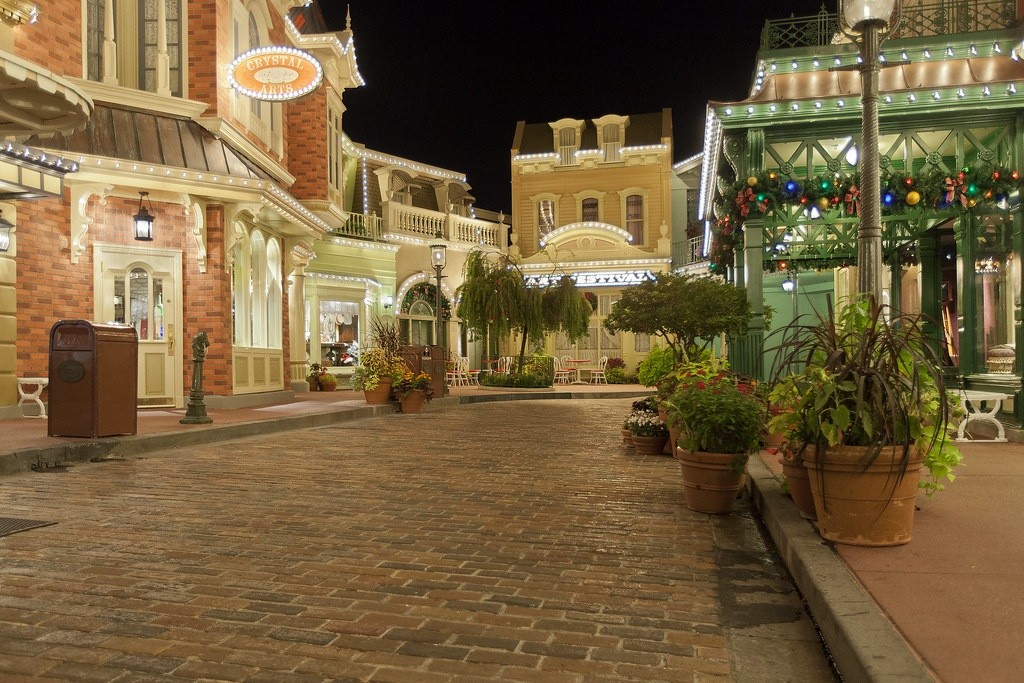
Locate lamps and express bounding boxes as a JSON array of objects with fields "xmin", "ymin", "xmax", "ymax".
[
  {"xmin": 782, "ymin": 271, "xmax": 794, "ymax": 294},
  {"xmin": 384, "ymin": 296, "xmax": 393, "ymax": 308},
  {"xmin": 132, "ymin": 191, "xmax": 156, "ymax": 242},
  {"xmin": 0, "ymin": 209, "xmax": 16, "ymax": 253}
]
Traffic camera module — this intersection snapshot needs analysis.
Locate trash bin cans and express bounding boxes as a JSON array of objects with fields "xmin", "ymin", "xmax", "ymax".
[
  {"xmin": 399, "ymin": 345, "xmax": 445, "ymax": 398},
  {"xmin": 48, "ymin": 320, "xmax": 138, "ymax": 438}
]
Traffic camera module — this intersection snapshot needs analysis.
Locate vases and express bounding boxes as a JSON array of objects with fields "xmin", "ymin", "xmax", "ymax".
[
  {"xmin": 632, "ymin": 435, "xmax": 668, "ymax": 454},
  {"xmin": 622, "ymin": 429, "xmax": 635, "ymax": 448},
  {"xmin": 676, "ymin": 446, "xmax": 751, "ymax": 514},
  {"xmin": 401, "ymin": 390, "xmax": 427, "ymax": 414}
]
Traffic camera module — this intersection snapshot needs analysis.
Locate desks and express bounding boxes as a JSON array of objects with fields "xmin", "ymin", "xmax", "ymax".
[
  {"xmin": 443, "ymin": 360, "xmax": 452, "ymax": 364},
  {"xmin": 480, "ymin": 359, "xmax": 500, "ymax": 375},
  {"xmin": 567, "ymin": 360, "xmax": 592, "ymax": 385}
]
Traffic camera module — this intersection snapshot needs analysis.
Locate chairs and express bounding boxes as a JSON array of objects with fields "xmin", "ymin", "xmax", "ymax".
[
  {"xmin": 553, "ymin": 356, "xmax": 609, "ymax": 385},
  {"xmin": 445, "ymin": 352, "xmax": 513, "ymax": 388}
]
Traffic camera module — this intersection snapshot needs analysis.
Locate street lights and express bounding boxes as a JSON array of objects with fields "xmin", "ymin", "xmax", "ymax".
[
  {"xmin": 827, "ymin": 0, "xmax": 912, "ymax": 325},
  {"xmin": 429, "ymin": 231, "xmax": 448, "ymax": 395}
]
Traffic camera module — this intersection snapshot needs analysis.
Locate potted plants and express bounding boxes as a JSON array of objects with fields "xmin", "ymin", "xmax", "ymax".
[
  {"xmin": 306, "ymin": 363, "xmax": 337, "ymax": 392},
  {"xmin": 601, "ymin": 269, "xmax": 779, "ymax": 451},
  {"xmin": 349, "ymin": 316, "xmax": 426, "ymax": 403},
  {"xmin": 747, "ymin": 292, "xmax": 966, "ymax": 546}
]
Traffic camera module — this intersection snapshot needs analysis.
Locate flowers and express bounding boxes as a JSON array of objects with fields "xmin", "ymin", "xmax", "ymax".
[
  {"xmin": 622, "ymin": 398, "xmax": 668, "ymax": 437},
  {"xmin": 392, "ymin": 368, "xmax": 435, "ymax": 405},
  {"xmin": 662, "ymin": 370, "xmax": 775, "ymax": 484}
]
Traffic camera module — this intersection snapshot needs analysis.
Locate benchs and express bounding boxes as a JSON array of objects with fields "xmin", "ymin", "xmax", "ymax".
[
  {"xmin": 947, "ymin": 388, "xmax": 1008, "ymax": 445},
  {"xmin": 17, "ymin": 377, "xmax": 49, "ymax": 420}
]
